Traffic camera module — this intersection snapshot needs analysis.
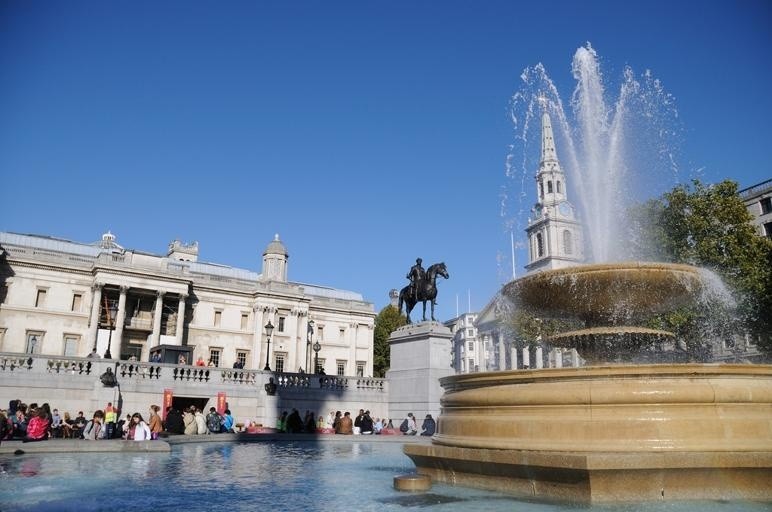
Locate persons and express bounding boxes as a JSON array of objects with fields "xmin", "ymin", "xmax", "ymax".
[
  {"xmin": 276, "ymin": 406, "xmax": 436, "ymax": 438},
  {"xmin": 104, "ymin": 349, "xmax": 112, "ymax": 358},
  {"xmin": 0, "ymin": 398, "xmax": 236, "ymax": 442},
  {"xmin": 87, "ymin": 348, "xmax": 101, "ymax": 358},
  {"xmin": 233, "ymin": 358, "xmax": 244, "ymax": 369},
  {"xmin": 179, "ymin": 354, "xmax": 187, "ymax": 364},
  {"xmin": 264, "ymin": 377, "xmax": 277, "ymax": 396},
  {"xmin": 100, "ymin": 367, "xmax": 117, "ymax": 387},
  {"xmin": 150, "ymin": 351, "xmax": 163, "ymax": 362},
  {"xmin": 319, "ymin": 368, "xmax": 327, "ymax": 374},
  {"xmin": 207, "ymin": 357, "xmax": 216, "ymax": 367},
  {"xmin": 128, "ymin": 352, "xmax": 138, "ymax": 361},
  {"xmin": 195, "ymin": 355, "xmax": 206, "ymax": 366},
  {"xmin": 406, "ymin": 257, "xmax": 440, "ymax": 306}
]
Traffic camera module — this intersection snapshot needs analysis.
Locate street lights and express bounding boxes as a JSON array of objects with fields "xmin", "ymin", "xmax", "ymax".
[
  {"xmin": 310, "ymin": 320, "xmax": 315, "ymax": 374},
  {"xmin": 313, "ymin": 341, "xmax": 321, "ymax": 374},
  {"xmin": 264, "ymin": 321, "xmax": 274, "ymax": 370}
]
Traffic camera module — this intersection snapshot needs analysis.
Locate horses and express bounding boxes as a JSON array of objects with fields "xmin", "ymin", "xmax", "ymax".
[{"xmin": 398, "ymin": 262, "xmax": 450, "ymax": 325}]
